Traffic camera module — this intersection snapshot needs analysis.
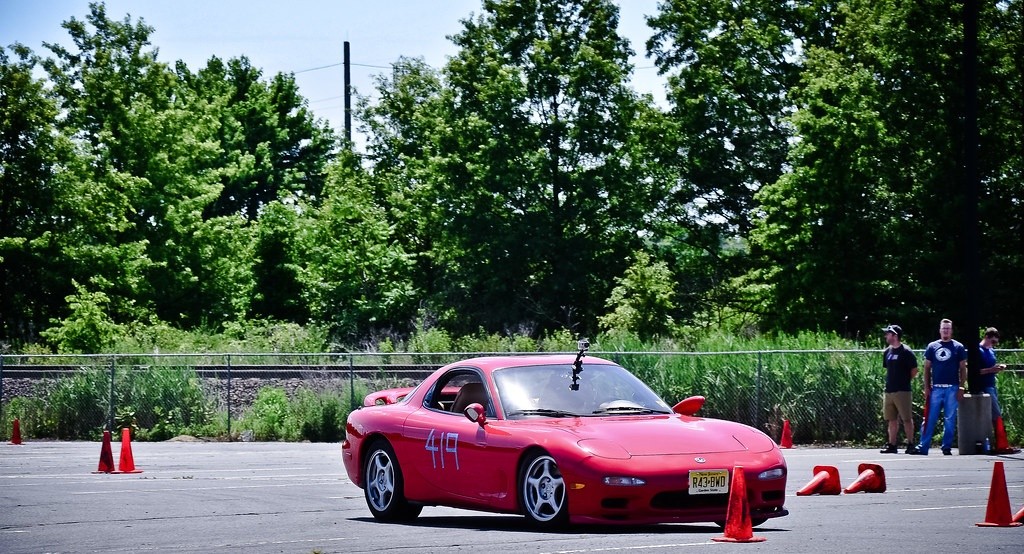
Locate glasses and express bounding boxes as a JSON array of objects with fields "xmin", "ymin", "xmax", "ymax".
[
  {"xmin": 987, "ymin": 337, "xmax": 999, "ymax": 345},
  {"xmin": 887, "ymin": 326, "xmax": 898, "ymax": 335}
]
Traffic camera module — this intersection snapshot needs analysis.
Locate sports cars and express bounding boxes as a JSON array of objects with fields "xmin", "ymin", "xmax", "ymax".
[{"xmin": 340, "ymin": 340, "xmax": 789, "ymax": 533}]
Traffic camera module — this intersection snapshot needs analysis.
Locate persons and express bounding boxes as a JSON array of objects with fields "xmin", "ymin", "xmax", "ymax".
[
  {"xmin": 979, "ymin": 326, "xmax": 1022, "ymax": 454},
  {"xmin": 915, "ymin": 319, "xmax": 968, "ymax": 456},
  {"xmin": 880, "ymin": 325, "xmax": 918, "ymax": 454}
]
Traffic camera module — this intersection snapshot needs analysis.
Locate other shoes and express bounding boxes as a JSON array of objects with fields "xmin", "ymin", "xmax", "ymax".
[
  {"xmin": 880, "ymin": 443, "xmax": 898, "ymax": 453},
  {"xmin": 910, "ymin": 446, "xmax": 928, "ymax": 455},
  {"xmin": 905, "ymin": 443, "xmax": 915, "ymax": 453},
  {"xmin": 941, "ymin": 448, "xmax": 953, "ymax": 455}
]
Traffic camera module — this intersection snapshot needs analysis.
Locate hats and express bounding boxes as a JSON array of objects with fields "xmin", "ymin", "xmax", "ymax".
[{"xmin": 882, "ymin": 325, "xmax": 903, "ymax": 337}]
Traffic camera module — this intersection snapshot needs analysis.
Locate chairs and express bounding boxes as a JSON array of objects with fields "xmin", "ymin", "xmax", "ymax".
[{"xmin": 450, "ymin": 382, "xmax": 488, "ymax": 415}]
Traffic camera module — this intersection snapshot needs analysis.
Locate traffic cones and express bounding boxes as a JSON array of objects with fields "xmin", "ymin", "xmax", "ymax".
[
  {"xmin": 7, "ymin": 418, "xmax": 26, "ymax": 445},
  {"xmin": 110, "ymin": 427, "xmax": 142, "ymax": 474},
  {"xmin": 975, "ymin": 461, "xmax": 1022, "ymax": 527},
  {"xmin": 796, "ymin": 465, "xmax": 841, "ymax": 496},
  {"xmin": 992, "ymin": 416, "xmax": 1013, "ymax": 454},
  {"xmin": 1011, "ymin": 507, "xmax": 1024, "ymax": 523},
  {"xmin": 844, "ymin": 464, "xmax": 888, "ymax": 494},
  {"xmin": 711, "ymin": 465, "xmax": 767, "ymax": 542},
  {"xmin": 777, "ymin": 419, "xmax": 797, "ymax": 449},
  {"xmin": 91, "ymin": 431, "xmax": 116, "ymax": 475}
]
